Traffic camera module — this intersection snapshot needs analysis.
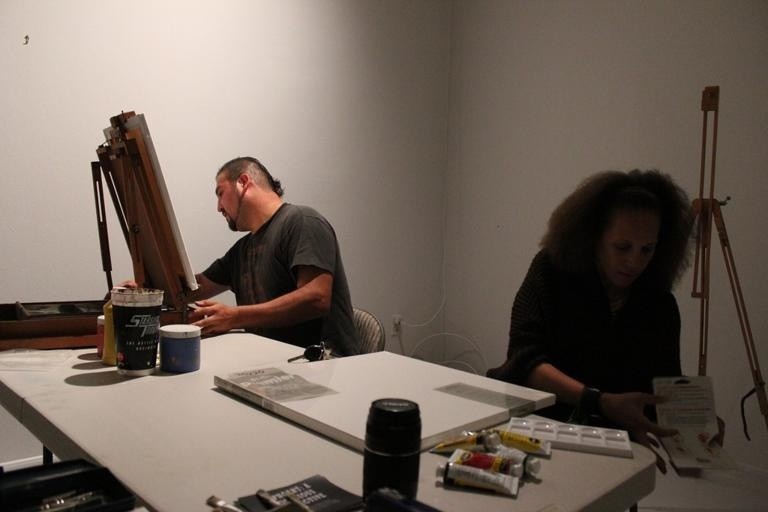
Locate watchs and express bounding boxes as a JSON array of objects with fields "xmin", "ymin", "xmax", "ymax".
[{"xmin": 579, "ymin": 383, "xmax": 604, "ymax": 423}]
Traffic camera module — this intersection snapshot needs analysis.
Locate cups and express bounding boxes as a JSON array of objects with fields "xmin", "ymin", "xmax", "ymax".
[{"xmin": 109, "ymin": 287, "xmax": 167, "ymax": 378}]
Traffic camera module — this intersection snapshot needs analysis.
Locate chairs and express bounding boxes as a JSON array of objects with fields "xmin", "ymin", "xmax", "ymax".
[{"xmin": 350, "ymin": 306, "xmax": 387, "ymax": 352}]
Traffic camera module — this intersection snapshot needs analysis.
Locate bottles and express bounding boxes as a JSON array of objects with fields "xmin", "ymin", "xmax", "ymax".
[
  {"xmin": 361, "ymin": 396, "xmax": 423, "ymax": 500},
  {"xmin": 159, "ymin": 323, "xmax": 201, "ymax": 374},
  {"xmin": 96, "ymin": 286, "xmax": 128, "ymax": 367}
]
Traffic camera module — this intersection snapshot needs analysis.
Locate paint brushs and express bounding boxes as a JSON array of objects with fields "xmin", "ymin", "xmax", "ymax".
[{"xmin": 207, "ymin": 488, "xmax": 312, "ymax": 512}]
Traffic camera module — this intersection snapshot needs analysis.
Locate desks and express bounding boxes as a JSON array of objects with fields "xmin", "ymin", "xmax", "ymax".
[{"xmin": 1, "ymin": 321, "xmax": 661, "ymax": 511}]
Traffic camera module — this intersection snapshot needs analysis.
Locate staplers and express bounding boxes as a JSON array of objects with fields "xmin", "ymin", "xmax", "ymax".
[{"xmin": 364, "ymin": 487, "xmax": 441, "ymax": 512}]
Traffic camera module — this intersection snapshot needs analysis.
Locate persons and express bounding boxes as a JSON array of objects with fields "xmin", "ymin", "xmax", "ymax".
[
  {"xmin": 484, "ymin": 166, "xmax": 689, "ymax": 474},
  {"xmin": 115, "ymin": 155, "xmax": 360, "ymax": 359}
]
